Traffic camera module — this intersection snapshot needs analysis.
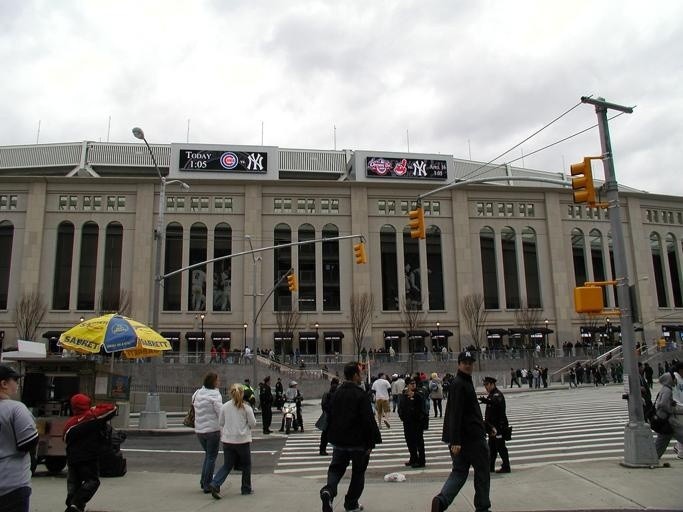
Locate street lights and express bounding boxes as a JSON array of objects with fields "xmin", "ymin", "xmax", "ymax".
[
  {"xmin": 436, "ymin": 321, "xmax": 440, "ymax": 351},
  {"xmin": 243, "ymin": 322, "xmax": 249, "ymax": 349},
  {"xmin": 314, "ymin": 321, "xmax": 320, "ymax": 365},
  {"xmin": 200, "ymin": 313, "xmax": 205, "ymax": 353},
  {"xmin": 606, "ymin": 317, "xmax": 611, "ymax": 342},
  {"xmin": 129, "ymin": 125, "xmax": 193, "ymax": 428},
  {"xmin": 241, "ymin": 234, "xmax": 264, "ymax": 388},
  {"xmin": 544, "ymin": 319, "xmax": 550, "ymax": 347},
  {"xmin": 79, "ymin": 316, "xmax": 85, "ymax": 322}
]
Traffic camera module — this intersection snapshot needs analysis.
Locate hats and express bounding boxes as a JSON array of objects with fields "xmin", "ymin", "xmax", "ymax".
[
  {"xmin": 482, "ymin": 376, "xmax": 498, "ymax": 385},
  {"xmin": 458, "ymin": 351, "xmax": 477, "ymax": 365},
  {"xmin": 0, "ymin": 365, "xmax": 25, "ymax": 381}
]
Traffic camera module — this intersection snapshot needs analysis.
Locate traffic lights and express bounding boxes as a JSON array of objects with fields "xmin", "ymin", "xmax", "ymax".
[
  {"xmin": 286, "ymin": 273, "xmax": 298, "ymax": 293},
  {"xmin": 353, "ymin": 242, "xmax": 367, "ymax": 264},
  {"xmin": 571, "ymin": 155, "xmax": 595, "ymax": 207},
  {"xmin": 407, "ymin": 207, "xmax": 426, "ymax": 240}
]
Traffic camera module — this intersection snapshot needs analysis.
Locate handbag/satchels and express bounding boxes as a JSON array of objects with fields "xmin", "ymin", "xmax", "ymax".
[
  {"xmin": 432, "ymin": 379, "xmax": 439, "ymax": 392},
  {"xmin": 650, "ymin": 419, "xmax": 675, "ymax": 435},
  {"xmin": 182, "ymin": 389, "xmax": 200, "ymax": 428}
]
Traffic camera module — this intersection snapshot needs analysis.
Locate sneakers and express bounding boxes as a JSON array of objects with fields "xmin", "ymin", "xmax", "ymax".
[
  {"xmin": 431, "ymin": 495, "xmax": 443, "ymax": 512},
  {"xmin": 495, "ymin": 467, "xmax": 511, "ymax": 474},
  {"xmin": 405, "ymin": 460, "xmax": 426, "ymax": 468},
  {"xmin": 345, "ymin": 503, "xmax": 363, "ymax": 512},
  {"xmin": 263, "ymin": 429, "xmax": 273, "ymax": 434},
  {"xmin": 384, "ymin": 420, "xmax": 391, "ymax": 428},
  {"xmin": 319, "ymin": 490, "xmax": 334, "ymax": 512},
  {"xmin": 208, "ymin": 483, "xmax": 223, "ymax": 499}
]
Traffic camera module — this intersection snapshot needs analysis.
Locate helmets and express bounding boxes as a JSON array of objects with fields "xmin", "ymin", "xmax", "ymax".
[{"xmin": 288, "ymin": 381, "xmax": 298, "ymax": 387}]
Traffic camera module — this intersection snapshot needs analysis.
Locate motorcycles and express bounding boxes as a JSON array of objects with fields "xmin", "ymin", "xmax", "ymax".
[{"xmin": 281, "ymin": 393, "xmax": 304, "ymax": 434}]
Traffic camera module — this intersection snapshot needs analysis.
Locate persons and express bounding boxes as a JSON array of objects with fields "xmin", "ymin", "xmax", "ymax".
[
  {"xmin": 636, "ymin": 336, "xmax": 683, "ymax": 459},
  {"xmin": 191, "ymin": 266, "xmax": 232, "ymax": 311},
  {"xmin": 62, "ymin": 394, "xmax": 118, "ymax": 512},
  {"xmin": 398, "ymin": 380, "xmax": 426, "ymax": 468},
  {"xmin": 477, "ymin": 376, "xmax": 511, "ymax": 473},
  {"xmin": 431, "ymin": 351, "xmax": 498, "ymax": 512},
  {"xmin": 563, "ymin": 336, "xmax": 624, "ymax": 388},
  {"xmin": 279, "ymin": 380, "xmax": 304, "ymax": 433},
  {"xmin": 260, "ymin": 376, "xmax": 273, "ymax": 434},
  {"xmin": 468, "ymin": 339, "xmax": 555, "ymax": 389},
  {"xmin": 243, "ymin": 379, "xmax": 256, "ymax": 412},
  {"xmin": 410, "ymin": 267, "xmax": 434, "ymax": 309},
  {"xmin": 209, "ymin": 345, "xmax": 300, "ymax": 372},
  {"xmin": 275, "ymin": 377, "xmax": 283, "ymax": 410},
  {"xmin": 315, "ymin": 379, "xmax": 340, "ymax": 455},
  {"xmin": 361, "ymin": 346, "xmax": 455, "ymax": 428},
  {"xmin": 209, "ymin": 383, "xmax": 256, "ymax": 499},
  {"xmin": 320, "ymin": 362, "xmax": 382, "ymax": 512},
  {"xmin": 191, "ymin": 373, "xmax": 223, "ymax": 493},
  {"xmin": 405, "ymin": 263, "xmax": 422, "ymax": 308},
  {"xmin": 0, "ymin": 365, "xmax": 39, "ymax": 512}
]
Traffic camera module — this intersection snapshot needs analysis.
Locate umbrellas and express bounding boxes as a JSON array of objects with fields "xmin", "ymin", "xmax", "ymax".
[{"xmin": 56, "ymin": 313, "xmax": 173, "ymax": 398}]
